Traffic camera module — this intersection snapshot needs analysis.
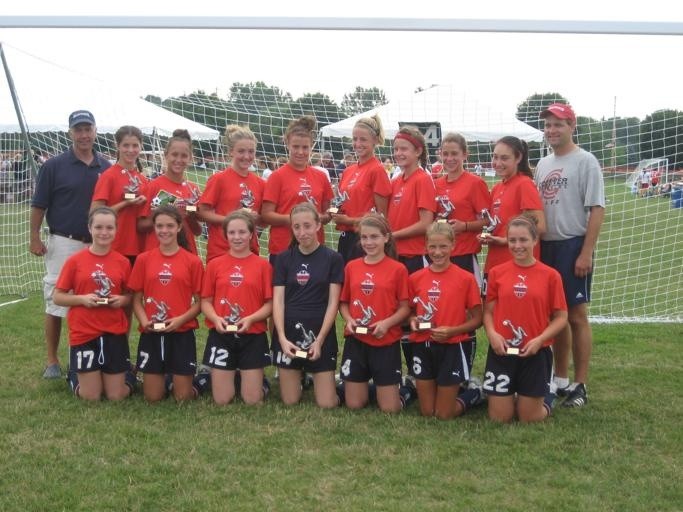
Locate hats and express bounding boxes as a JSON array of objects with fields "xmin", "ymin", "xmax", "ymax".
[
  {"xmin": 540, "ymin": 101, "xmax": 576, "ymax": 123},
  {"xmin": 67, "ymin": 109, "xmax": 96, "ymax": 128}
]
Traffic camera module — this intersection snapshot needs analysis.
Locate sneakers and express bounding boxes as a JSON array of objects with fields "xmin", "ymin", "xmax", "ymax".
[
  {"xmin": 42, "ymin": 360, "xmax": 62, "ymax": 379},
  {"xmin": 196, "ymin": 363, "xmax": 212, "ymax": 377},
  {"xmin": 129, "ymin": 362, "xmax": 139, "ymax": 377},
  {"xmin": 400, "ymin": 376, "xmax": 418, "ymax": 398},
  {"xmin": 467, "ymin": 376, "xmax": 488, "ymax": 407},
  {"xmin": 65, "ymin": 361, "xmax": 72, "ymax": 383},
  {"xmin": 555, "ymin": 382, "xmax": 589, "ymax": 409},
  {"xmin": 301, "ymin": 368, "xmax": 315, "ymax": 390}
]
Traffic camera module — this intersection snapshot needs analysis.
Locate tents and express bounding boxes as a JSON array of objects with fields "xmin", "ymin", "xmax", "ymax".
[
  {"xmin": 0, "ymin": 95, "xmax": 220, "ymax": 141},
  {"xmin": 318, "ymin": 84, "xmax": 544, "ymax": 142}
]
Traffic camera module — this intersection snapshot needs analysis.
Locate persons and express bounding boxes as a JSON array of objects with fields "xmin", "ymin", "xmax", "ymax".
[
  {"xmin": 29, "ymin": 110, "xmax": 112, "ymax": 378},
  {"xmin": 641, "ymin": 168, "xmax": 672, "ymax": 197},
  {"xmin": 535, "ymin": 104, "xmax": 604, "ymax": 408},
  {"xmin": 49, "ymin": 116, "xmax": 567, "ymax": 423},
  {"xmin": 0, "ymin": 149, "xmax": 47, "ymax": 204}
]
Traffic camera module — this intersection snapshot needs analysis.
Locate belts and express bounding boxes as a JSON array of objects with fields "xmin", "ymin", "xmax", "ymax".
[{"xmin": 47, "ymin": 228, "xmax": 93, "ymax": 246}]
